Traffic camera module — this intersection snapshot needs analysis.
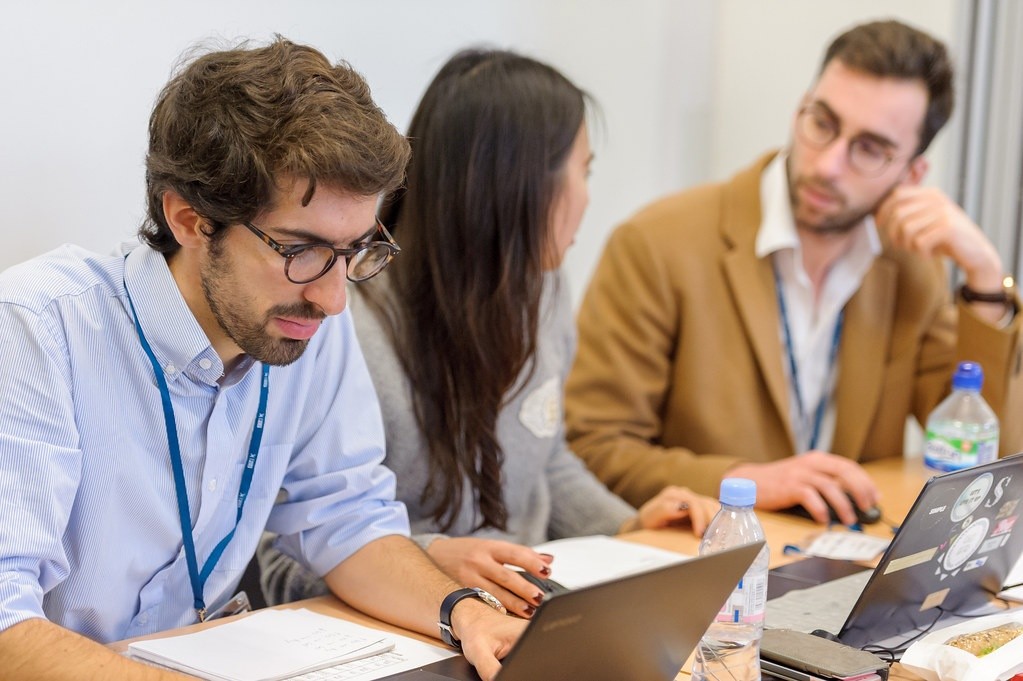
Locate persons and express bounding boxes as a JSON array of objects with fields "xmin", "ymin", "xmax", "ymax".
[
  {"xmin": 256, "ymin": 48, "xmax": 721, "ymax": 619},
  {"xmin": 560, "ymin": 20, "xmax": 1023, "ymax": 526},
  {"xmin": 0, "ymin": 38, "xmax": 532, "ymax": 681}
]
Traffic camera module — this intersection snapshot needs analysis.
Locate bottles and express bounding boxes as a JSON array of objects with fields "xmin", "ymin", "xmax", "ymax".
[
  {"xmin": 690, "ymin": 476, "xmax": 770, "ymax": 681},
  {"xmin": 920, "ymin": 361, "xmax": 1000, "ymax": 489}
]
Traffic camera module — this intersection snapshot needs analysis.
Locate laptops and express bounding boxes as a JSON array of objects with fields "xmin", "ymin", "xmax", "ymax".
[
  {"xmin": 762, "ymin": 452, "xmax": 1023, "ymax": 649},
  {"xmin": 375, "ymin": 540, "xmax": 768, "ymax": 681}
]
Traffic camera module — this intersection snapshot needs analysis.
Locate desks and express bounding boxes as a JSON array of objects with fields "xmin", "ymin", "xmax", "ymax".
[{"xmin": 106, "ymin": 447, "xmax": 1023, "ymax": 681}]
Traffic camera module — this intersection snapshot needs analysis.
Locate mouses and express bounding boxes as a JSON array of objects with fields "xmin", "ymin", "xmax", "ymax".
[
  {"xmin": 506, "ymin": 569, "xmax": 570, "ymax": 608},
  {"xmin": 783, "ymin": 490, "xmax": 881, "ymax": 527}
]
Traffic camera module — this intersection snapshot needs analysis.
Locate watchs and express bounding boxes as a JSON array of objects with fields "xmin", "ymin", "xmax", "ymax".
[
  {"xmin": 437, "ymin": 587, "xmax": 507, "ymax": 647},
  {"xmin": 959, "ymin": 274, "xmax": 1014, "ymax": 302}
]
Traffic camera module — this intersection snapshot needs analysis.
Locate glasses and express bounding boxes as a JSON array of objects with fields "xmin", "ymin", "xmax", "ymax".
[
  {"xmin": 795, "ymin": 107, "xmax": 893, "ymax": 180},
  {"xmin": 239, "ymin": 219, "xmax": 401, "ymax": 285}
]
